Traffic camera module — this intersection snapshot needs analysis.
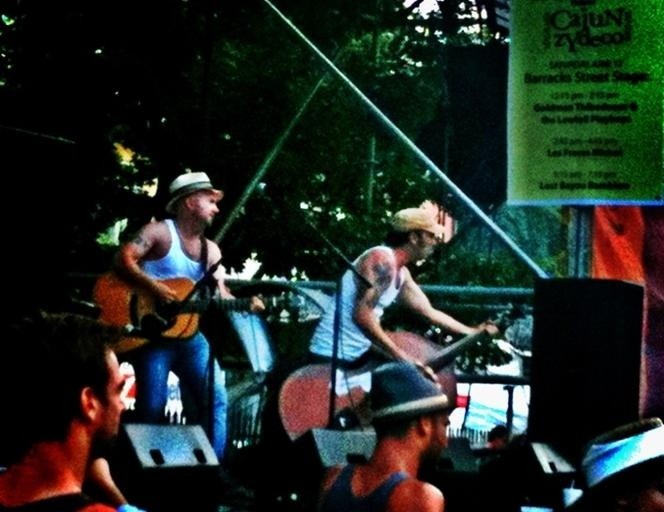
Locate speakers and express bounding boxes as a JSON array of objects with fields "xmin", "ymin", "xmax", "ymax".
[
  {"xmin": 527, "ymin": 276, "xmax": 648, "ymax": 510},
  {"xmin": 99, "ymin": 420, "xmax": 222, "ymax": 512},
  {"xmin": 288, "ymin": 428, "xmax": 380, "ymax": 501}
]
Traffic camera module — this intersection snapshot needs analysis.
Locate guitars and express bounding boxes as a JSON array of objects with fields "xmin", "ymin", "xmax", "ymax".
[
  {"xmin": 279, "ymin": 319, "xmax": 504, "ymax": 442},
  {"xmin": 96, "ymin": 275, "xmax": 278, "ymax": 352}
]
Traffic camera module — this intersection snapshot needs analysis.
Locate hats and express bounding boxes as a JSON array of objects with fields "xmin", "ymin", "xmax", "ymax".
[
  {"xmin": 390, "ymin": 199, "xmax": 455, "ymax": 244},
  {"xmin": 165, "ymin": 171, "xmax": 224, "ymax": 212},
  {"xmin": 364, "ymin": 360, "xmax": 450, "ymax": 419}
]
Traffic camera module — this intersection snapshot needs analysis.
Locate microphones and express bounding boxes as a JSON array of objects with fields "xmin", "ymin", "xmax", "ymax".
[{"xmin": 254, "ymin": 181, "xmax": 297, "ymax": 216}]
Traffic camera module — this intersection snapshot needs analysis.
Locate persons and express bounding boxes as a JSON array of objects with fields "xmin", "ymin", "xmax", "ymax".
[
  {"xmin": 313, "ymin": 359, "xmax": 451, "ymax": 512},
  {"xmin": 0, "ymin": 311, "xmax": 150, "ymax": 512},
  {"xmin": 478, "ymin": 424, "xmax": 513, "ymax": 470},
  {"xmin": 108, "ymin": 170, "xmax": 267, "ymax": 462},
  {"xmin": 307, "ymin": 208, "xmax": 500, "ymax": 392}
]
